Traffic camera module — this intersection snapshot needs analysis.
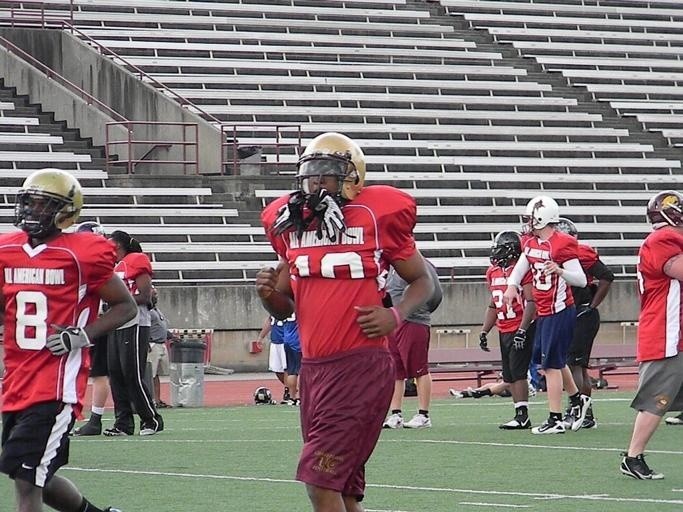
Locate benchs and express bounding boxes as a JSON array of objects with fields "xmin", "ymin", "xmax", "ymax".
[{"xmin": 427, "ymin": 344, "xmax": 640, "ymax": 390}]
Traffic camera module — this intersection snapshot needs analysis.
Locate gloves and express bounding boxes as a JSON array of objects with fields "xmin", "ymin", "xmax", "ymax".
[
  {"xmin": 273, "ymin": 188, "xmax": 345, "ymax": 242},
  {"xmin": 46, "ymin": 324, "xmax": 90, "ymax": 355},
  {"xmin": 480, "ymin": 332, "xmax": 490, "ymax": 351},
  {"xmin": 513, "ymin": 328, "xmax": 526, "ymax": 351}
]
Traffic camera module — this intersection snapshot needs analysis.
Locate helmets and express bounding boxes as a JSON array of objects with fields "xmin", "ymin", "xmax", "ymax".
[
  {"xmin": 255, "ymin": 387, "xmax": 271, "ymax": 405},
  {"xmin": 490, "ymin": 197, "xmax": 578, "ymax": 263},
  {"xmin": 297, "ymin": 132, "xmax": 366, "ymax": 201},
  {"xmin": 19, "ymin": 168, "xmax": 105, "ymax": 235},
  {"xmin": 647, "ymin": 190, "xmax": 683, "ymax": 230}
]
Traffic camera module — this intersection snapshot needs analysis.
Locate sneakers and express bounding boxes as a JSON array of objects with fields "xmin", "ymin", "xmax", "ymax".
[
  {"xmin": 665, "ymin": 415, "xmax": 683, "ymax": 424},
  {"xmin": 281, "ymin": 395, "xmax": 300, "ymax": 406},
  {"xmin": 449, "ymin": 387, "xmax": 476, "ymax": 398},
  {"xmin": 382, "ymin": 412, "xmax": 431, "ymax": 429},
  {"xmin": 69, "ymin": 423, "xmax": 155, "ymax": 436},
  {"xmin": 153, "ymin": 400, "xmax": 173, "ymax": 408},
  {"xmin": 499, "ymin": 394, "xmax": 595, "ymax": 434},
  {"xmin": 620, "ymin": 452, "xmax": 664, "ymax": 479}
]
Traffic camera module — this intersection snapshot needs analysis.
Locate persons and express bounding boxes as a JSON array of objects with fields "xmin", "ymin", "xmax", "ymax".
[
  {"xmin": 0, "ymin": 166, "xmax": 138, "ymax": 512},
  {"xmin": 383, "ymin": 255, "xmax": 443, "ymax": 429},
  {"xmin": 620, "ymin": 189, "xmax": 683, "ymax": 482},
  {"xmin": 257, "ymin": 309, "xmax": 303, "ymax": 407},
  {"xmin": 450, "ymin": 362, "xmax": 608, "ymax": 398},
  {"xmin": 253, "ymin": 129, "xmax": 436, "ymax": 511},
  {"xmin": 478, "ymin": 193, "xmax": 616, "ymax": 435},
  {"xmin": 66, "ymin": 220, "xmax": 174, "ymax": 438}
]
